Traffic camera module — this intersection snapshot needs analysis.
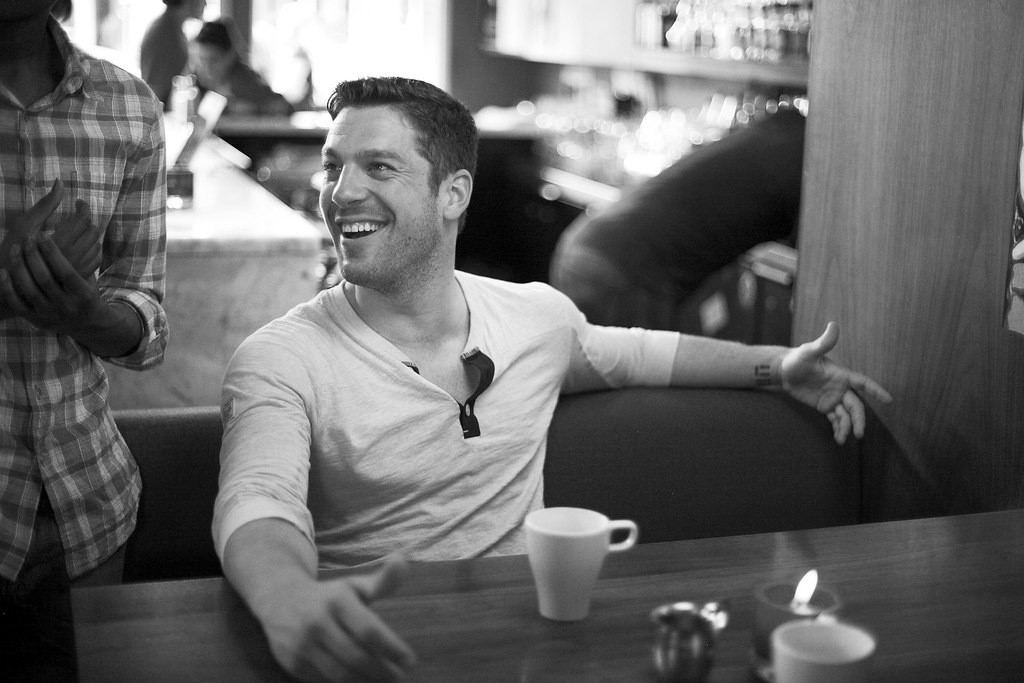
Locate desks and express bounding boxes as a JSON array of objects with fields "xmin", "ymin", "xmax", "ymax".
[{"xmin": 71, "ymin": 505, "xmax": 1024, "ymax": 683}]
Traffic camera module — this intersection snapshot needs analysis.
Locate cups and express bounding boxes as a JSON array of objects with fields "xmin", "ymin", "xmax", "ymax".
[
  {"xmin": 645, "ymin": 599, "xmax": 729, "ymax": 683},
  {"xmin": 768, "ymin": 611, "xmax": 878, "ymax": 682},
  {"xmin": 747, "ymin": 584, "xmax": 842, "ymax": 676},
  {"xmin": 167, "ymin": 168, "xmax": 193, "ymax": 210},
  {"xmin": 522, "ymin": 506, "xmax": 638, "ymax": 621}
]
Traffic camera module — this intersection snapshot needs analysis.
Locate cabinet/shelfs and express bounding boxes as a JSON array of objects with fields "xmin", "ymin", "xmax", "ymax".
[{"xmin": 485, "ymin": 38, "xmax": 810, "ymax": 293}]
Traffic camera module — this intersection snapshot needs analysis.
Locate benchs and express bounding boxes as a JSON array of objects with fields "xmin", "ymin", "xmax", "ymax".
[{"xmin": 108, "ymin": 389, "xmax": 941, "ymax": 582}]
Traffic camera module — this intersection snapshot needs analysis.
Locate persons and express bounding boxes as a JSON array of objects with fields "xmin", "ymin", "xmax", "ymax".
[
  {"xmin": 0, "ymin": 0, "xmax": 172, "ymax": 683},
  {"xmin": 139, "ymin": 0, "xmax": 207, "ymax": 112},
  {"xmin": 210, "ymin": 77, "xmax": 895, "ymax": 683},
  {"xmin": 555, "ymin": 106, "xmax": 805, "ymax": 338},
  {"xmin": 197, "ymin": 17, "xmax": 294, "ymax": 116}
]
